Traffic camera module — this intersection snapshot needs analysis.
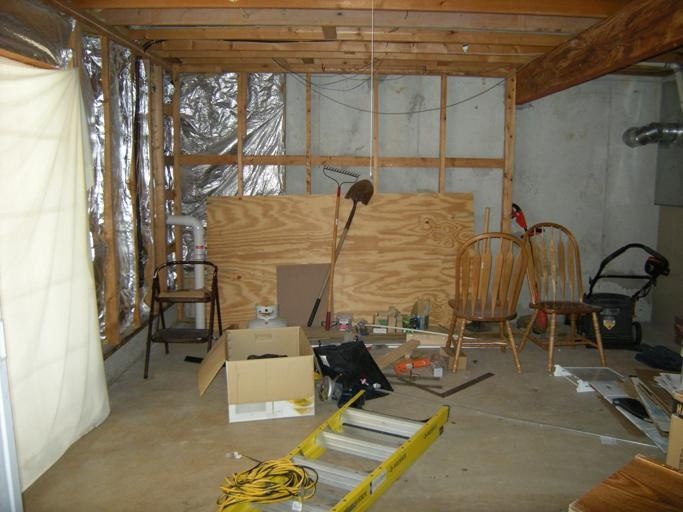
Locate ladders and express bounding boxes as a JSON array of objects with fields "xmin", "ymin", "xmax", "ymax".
[{"xmin": 218, "ymin": 388, "xmax": 451, "ymax": 512}]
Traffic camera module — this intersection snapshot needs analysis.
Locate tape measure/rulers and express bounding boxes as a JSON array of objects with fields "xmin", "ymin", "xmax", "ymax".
[{"xmin": 383, "ymin": 373, "xmax": 495, "ymax": 398}]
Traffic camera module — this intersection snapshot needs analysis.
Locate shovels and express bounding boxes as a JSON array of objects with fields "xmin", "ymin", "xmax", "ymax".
[{"xmin": 307, "ymin": 179, "xmax": 374, "ymax": 327}]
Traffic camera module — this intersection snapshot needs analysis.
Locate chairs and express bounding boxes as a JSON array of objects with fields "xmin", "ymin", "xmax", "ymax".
[
  {"xmin": 517, "ymin": 222, "xmax": 606, "ymax": 377},
  {"xmin": 143, "ymin": 261, "xmax": 226, "ymax": 379},
  {"xmin": 446, "ymin": 232, "xmax": 529, "ymax": 374}
]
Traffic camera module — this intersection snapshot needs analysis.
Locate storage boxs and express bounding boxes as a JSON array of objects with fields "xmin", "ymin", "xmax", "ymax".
[{"xmin": 197, "ymin": 326, "xmax": 323, "ymax": 423}]
